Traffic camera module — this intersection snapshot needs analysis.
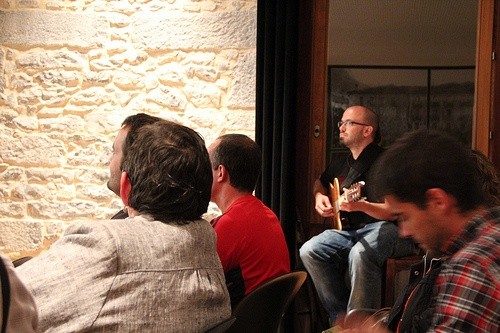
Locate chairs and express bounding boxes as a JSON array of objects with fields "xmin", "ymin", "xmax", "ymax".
[{"xmin": 231, "ymin": 271, "xmax": 308, "ymax": 333}]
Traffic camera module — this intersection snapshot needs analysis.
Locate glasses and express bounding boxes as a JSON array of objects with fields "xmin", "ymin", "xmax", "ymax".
[{"xmin": 337, "ymin": 121, "xmax": 368, "ymax": 127}]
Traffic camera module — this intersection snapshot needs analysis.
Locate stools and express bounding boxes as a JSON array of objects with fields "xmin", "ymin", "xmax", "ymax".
[{"xmin": 338, "ymin": 254, "xmax": 424, "ymax": 309}]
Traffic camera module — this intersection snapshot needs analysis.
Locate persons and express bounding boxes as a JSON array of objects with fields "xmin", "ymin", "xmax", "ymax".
[
  {"xmin": 335, "ymin": 129, "xmax": 500, "ymax": 333},
  {"xmin": 300, "ymin": 104, "xmax": 407, "ymax": 333},
  {"xmin": 206, "ymin": 134, "xmax": 290, "ymax": 318},
  {"xmin": 386, "ymin": 149, "xmax": 490, "ymax": 333},
  {"xmin": 16, "ymin": 121, "xmax": 231, "ymax": 333},
  {"xmin": 0, "ymin": 253, "xmax": 39, "ymax": 333},
  {"xmin": 12, "ymin": 112, "xmax": 171, "ymax": 268}
]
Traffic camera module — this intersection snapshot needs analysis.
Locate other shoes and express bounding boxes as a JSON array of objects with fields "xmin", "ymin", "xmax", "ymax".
[{"xmin": 322, "ymin": 325, "xmax": 343, "ymax": 333}]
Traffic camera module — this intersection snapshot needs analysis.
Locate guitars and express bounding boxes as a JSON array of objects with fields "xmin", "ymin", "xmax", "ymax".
[{"xmin": 325, "ymin": 176, "xmax": 367, "ymax": 231}]
